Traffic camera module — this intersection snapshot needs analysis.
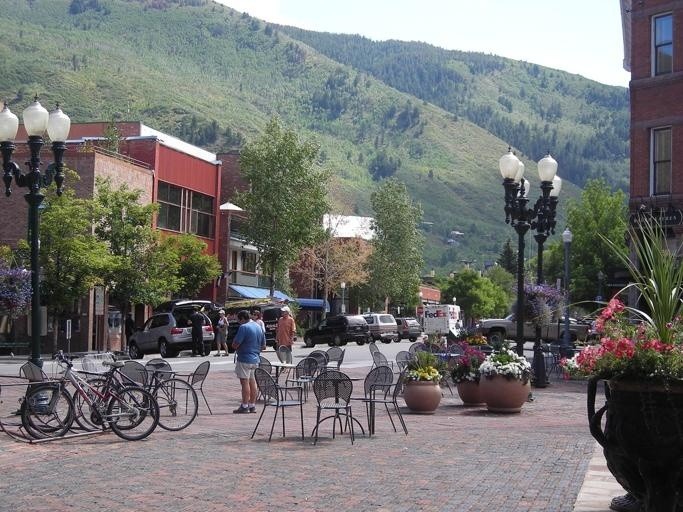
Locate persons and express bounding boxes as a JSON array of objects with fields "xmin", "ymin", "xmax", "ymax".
[
  {"xmin": 454, "ymin": 319, "xmax": 462, "ymax": 336},
  {"xmin": 250, "ymin": 310, "xmax": 266, "ymax": 334},
  {"xmin": 232, "ymin": 310, "xmax": 266, "ymax": 414},
  {"xmin": 213, "ymin": 309, "xmax": 229, "ymax": 356},
  {"xmin": 124, "ymin": 312, "xmax": 135, "ymax": 349},
  {"xmin": 275, "ymin": 305, "xmax": 296, "ymax": 374},
  {"xmin": 187, "ymin": 307, "xmax": 206, "ymax": 357}
]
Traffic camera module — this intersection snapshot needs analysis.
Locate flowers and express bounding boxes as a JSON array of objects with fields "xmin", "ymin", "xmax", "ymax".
[
  {"xmin": 450, "ymin": 346, "xmax": 530, "ymax": 384},
  {"xmin": 557, "ymin": 206, "xmax": 678, "ymax": 380},
  {"xmin": 2, "ymin": 259, "xmax": 33, "ymax": 318}
]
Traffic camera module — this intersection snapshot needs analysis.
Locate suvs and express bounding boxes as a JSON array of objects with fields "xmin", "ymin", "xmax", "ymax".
[{"xmin": 395, "ymin": 319, "xmax": 420, "ymax": 342}]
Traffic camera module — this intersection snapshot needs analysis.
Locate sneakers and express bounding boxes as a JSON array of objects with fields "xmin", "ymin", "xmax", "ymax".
[
  {"xmin": 233, "ymin": 404, "xmax": 249, "ymax": 413},
  {"xmin": 250, "ymin": 406, "xmax": 256, "ymax": 412}
]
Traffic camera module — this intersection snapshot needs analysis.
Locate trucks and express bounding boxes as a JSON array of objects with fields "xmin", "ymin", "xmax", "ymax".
[{"xmin": 422, "ymin": 302, "xmax": 466, "ymax": 338}]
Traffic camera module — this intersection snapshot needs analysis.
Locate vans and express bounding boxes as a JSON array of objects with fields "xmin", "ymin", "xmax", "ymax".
[
  {"xmin": 205, "ymin": 305, "xmax": 281, "ymax": 349},
  {"xmin": 364, "ymin": 314, "xmax": 398, "ymax": 343}
]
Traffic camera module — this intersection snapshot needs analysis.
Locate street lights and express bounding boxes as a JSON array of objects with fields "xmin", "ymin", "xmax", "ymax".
[
  {"xmin": 558, "ymin": 220, "xmax": 575, "ymax": 356},
  {"xmin": 499, "ymin": 145, "xmax": 561, "ymax": 357},
  {"xmin": 1, "ymin": 93, "xmax": 70, "ymax": 362}
]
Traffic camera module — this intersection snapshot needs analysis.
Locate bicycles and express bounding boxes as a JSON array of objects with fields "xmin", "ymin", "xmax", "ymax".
[{"xmin": 20, "ymin": 350, "xmax": 199, "ymax": 440}]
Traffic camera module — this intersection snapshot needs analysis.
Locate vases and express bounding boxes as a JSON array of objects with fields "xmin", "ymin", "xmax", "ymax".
[
  {"xmin": 582, "ymin": 370, "xmax": 683, "ymax": 511},
  {"xmin": 455, "ymin": 374, "xmax": 532, "ymax": 413}
]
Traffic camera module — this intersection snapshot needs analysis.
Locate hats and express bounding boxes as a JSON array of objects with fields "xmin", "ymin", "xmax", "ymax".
[
  {"xmin": 218, "ymin": 310, "xmax": 225, "ymax": 314},
  {"xmin": 280, "ymin": 306, "xmax": 290, "ymax": 311}
]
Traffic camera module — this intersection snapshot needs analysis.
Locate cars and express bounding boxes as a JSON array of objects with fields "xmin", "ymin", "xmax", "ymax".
[
  {"xmin": 304, "ymin": 315, "xmax": 369, "ymax": 346},
  {"xmin": 127, "ymin": 297, "xmax": 216, "ymax": 357}
]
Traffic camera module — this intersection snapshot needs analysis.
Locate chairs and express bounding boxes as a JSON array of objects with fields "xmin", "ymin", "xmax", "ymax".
[
  {"xmin": 72, "ymin": 352, "xmax": 211, "ymax": 427},
  {"xmin": 250, "ymin": 338, "xmax": 465, "ymax": 445}
]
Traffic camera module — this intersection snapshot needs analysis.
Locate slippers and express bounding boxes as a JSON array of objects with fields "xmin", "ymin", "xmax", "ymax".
[{"xmin": 213, "ymin": 354, "xmax": 229, "ymax": 356}]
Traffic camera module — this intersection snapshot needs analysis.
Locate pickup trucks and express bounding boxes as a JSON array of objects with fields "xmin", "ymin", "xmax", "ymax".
[{"xmin": 477, "ymin": 309, "xmax": 587, "ymax": 347}]
